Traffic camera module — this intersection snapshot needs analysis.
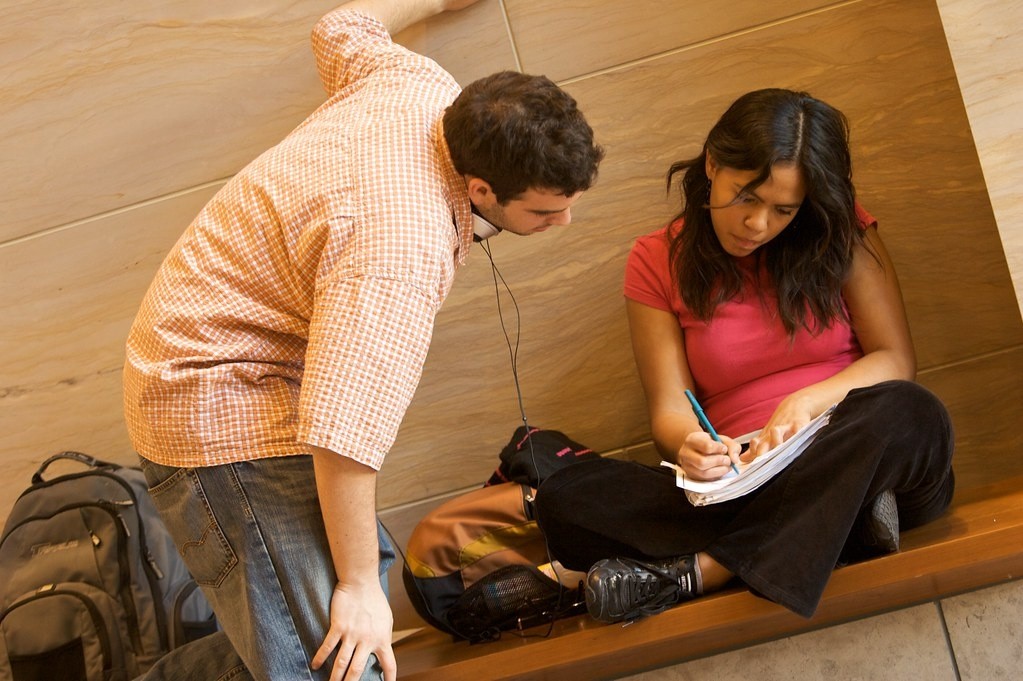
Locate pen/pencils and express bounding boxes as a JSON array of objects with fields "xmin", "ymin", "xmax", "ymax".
[{"xmin": 682, "ymin": 386, "xmax": 741, "ymax": 479}]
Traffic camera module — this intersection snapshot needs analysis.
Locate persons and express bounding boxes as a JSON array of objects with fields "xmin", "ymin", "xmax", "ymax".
[
  {"xmin": 533, "ymin": 85, "xmax": 956, "ymax": 625},
  {"xmin": 121, "ymin": 0, "xmax": 605, "ymax": 681}
]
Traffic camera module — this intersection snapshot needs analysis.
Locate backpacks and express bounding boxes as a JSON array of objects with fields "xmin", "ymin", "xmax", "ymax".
[
  {"xmin": 0, "ymin": 450, "xmax": 220, "ymax": 681},
  {"xmin": 401, "ymin": 476, "xmax": 585, "ymax": 639}
]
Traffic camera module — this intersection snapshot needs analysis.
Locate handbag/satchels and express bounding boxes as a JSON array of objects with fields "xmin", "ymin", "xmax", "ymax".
[{"xmin": 482, "ymin": 427, "xmax": 602, "ymax": 490}]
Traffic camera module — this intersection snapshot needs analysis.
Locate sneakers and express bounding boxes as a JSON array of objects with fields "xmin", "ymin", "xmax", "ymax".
[
  {"xmin": 583, "ymin": 554, "xmax": 695, "ymax": 628},
  {"xmin": 851, "ymin": 490, "xmax": 899, "ymax": 560}
]
